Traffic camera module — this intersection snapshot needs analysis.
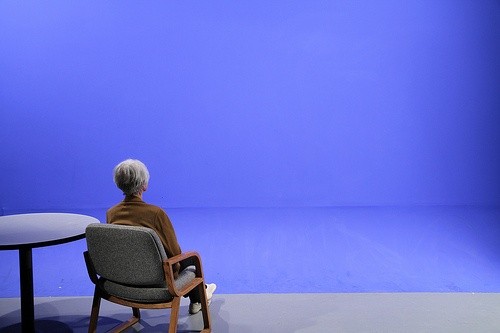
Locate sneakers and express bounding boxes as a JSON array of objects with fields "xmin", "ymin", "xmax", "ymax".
[
  {"xmin": 205, "ymin": 283, "xmax": 216, "ymax": 299},
  {"xmin": 188, "ymin": 299, "xmax": 211, "ymax": 314}
]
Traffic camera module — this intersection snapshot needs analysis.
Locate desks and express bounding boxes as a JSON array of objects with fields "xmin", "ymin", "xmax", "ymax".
[{"xmin": 0, "ymin": 212, "xmax": 104, "ymax": 333}]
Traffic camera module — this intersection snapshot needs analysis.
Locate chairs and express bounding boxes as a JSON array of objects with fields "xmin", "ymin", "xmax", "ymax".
[{"xmin": 82, "ymin": 222, "xmax": 212, "ymax": 333}]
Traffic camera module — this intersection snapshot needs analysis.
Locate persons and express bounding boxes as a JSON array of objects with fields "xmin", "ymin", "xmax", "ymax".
[{"xmin": 106, "ymin": 159, "xmax": 217, "ymax": 315}]
